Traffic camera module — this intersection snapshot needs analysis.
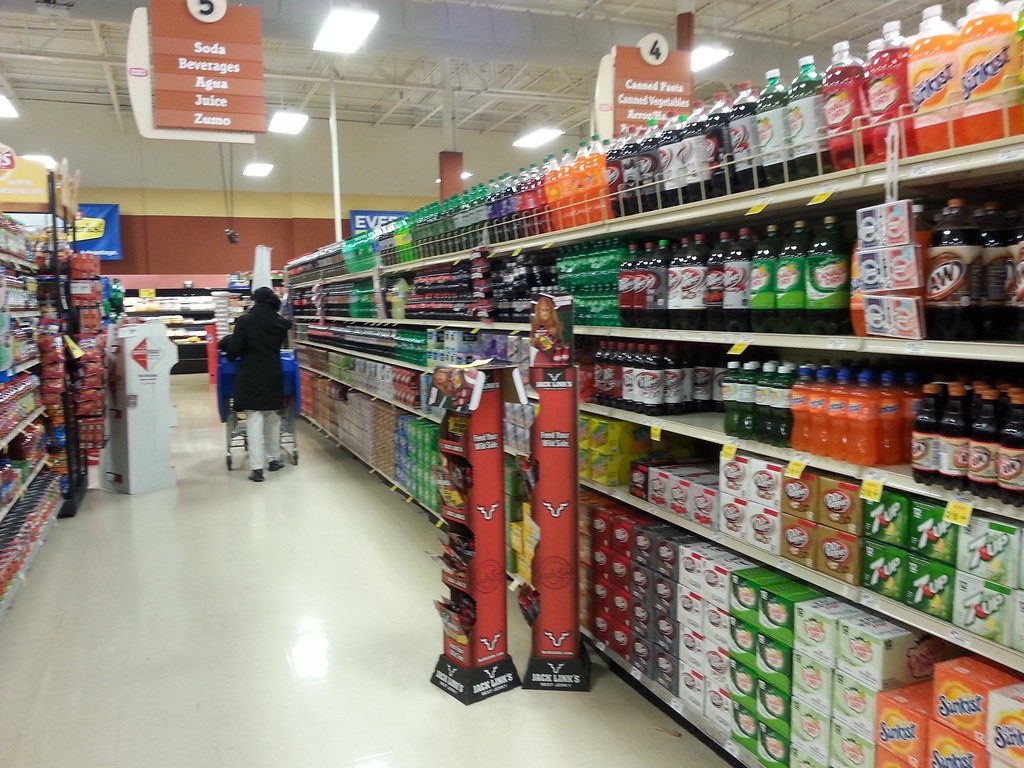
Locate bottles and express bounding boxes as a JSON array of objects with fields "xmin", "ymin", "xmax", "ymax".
[{"xmin": 282, "ymin": 0, "xmax": 1024, "ymax": 511}]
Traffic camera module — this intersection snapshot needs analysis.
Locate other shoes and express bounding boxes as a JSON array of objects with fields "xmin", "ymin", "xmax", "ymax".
[
  {"xmin": 269, "ymin": 460, "xmax": 284, "ymax": 471},
  {"xmin": 248, "ymin": 469, "xmax": 264, "ymax": 481}
]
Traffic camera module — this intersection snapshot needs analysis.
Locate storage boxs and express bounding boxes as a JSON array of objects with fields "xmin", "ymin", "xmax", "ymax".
[{"xmin": 293, "ymin": 346, "xmax": 1024, "ymax": 767}]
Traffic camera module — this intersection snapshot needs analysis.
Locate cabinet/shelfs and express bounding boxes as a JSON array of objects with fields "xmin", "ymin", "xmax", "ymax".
[
  {"xmin": 283, "ymin": 138, "xmax": 1024, "ymax": 767},
  {"xmin": 0, "ymin": 253, "xmax": 90, "ymax": 521},
  {"xmin": 123, "ymin": 308, "xmax": 217, "ymax": 375}
]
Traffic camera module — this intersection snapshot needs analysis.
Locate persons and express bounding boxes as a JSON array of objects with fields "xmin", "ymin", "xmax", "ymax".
[{"xmin": 224, "ymin": 286, "xmax": 294, "ymax": 483}]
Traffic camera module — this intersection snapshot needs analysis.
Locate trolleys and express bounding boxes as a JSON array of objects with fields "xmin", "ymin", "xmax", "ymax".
[{"xmin": 217, "ymin": 346, "xmax": 302, "ymax": 471}]
